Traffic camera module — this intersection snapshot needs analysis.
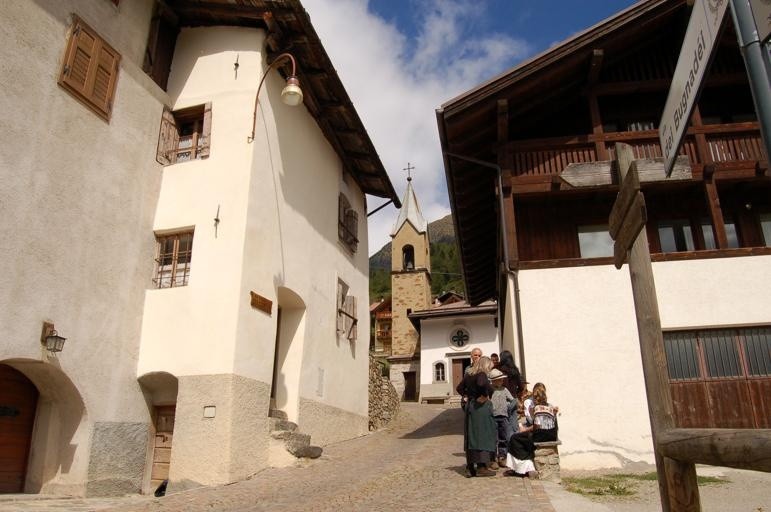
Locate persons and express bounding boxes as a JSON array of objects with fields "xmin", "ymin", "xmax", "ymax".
[{"xmin": 456, "ymin": 347, "xmax": 557, "ymax": 479}]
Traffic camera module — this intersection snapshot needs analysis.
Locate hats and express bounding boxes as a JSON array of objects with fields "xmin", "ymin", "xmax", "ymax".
[{"xmin": 487, "ymin": 369, "xmax": 508, "ymax": 379}]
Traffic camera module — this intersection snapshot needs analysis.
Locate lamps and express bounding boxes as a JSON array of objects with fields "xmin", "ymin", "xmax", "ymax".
[
  {"xmin": 252, "ymin": 53, "xmax": 303, "ymax": 139},
  {"xmin": 40, "ymin": 322, "xmax": 66, "ymax": 355}
]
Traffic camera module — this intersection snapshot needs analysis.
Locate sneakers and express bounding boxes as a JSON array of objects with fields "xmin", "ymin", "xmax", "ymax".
[{"xmin": 464, "ymin": 455, "xmax": 517, "ymax": 478}]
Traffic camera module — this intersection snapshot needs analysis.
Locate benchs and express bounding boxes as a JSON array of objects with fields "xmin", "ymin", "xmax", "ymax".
[{"xmin": 534, "ymin": 440, "xmax": 563, "ymax": 483}]
